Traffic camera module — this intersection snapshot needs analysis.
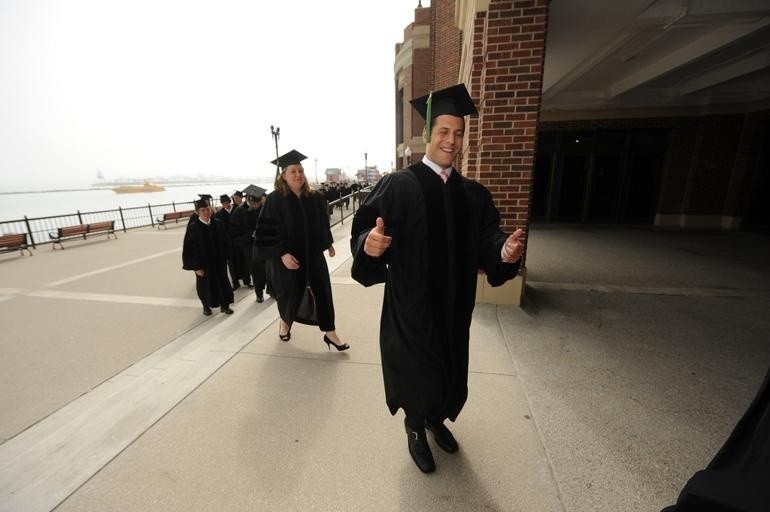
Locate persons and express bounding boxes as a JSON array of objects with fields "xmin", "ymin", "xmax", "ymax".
[
  {"xmin": 345, "ymin": 82, "xmax": 524, "ymax": 473},
  {"xmin": 256, "ymin": 150, "xmax": 350, "ymax": 353},
  {"xmin": 183, "ymin": 182, "xmax": 374, "ymax": 314},
  {"xmin": 658, "ymin": 372, "xmax": 769, "ymax": 511}
]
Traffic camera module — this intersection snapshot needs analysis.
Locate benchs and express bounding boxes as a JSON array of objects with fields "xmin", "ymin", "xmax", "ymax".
[
  {"xmin": 0, "ymin": 233, "xmax": 33, "ymax": 256},
  {"xmin": 158, "ymin": 211, "xmax": 194, "ymax": 230},
  {"xmin": 49, "ymin": 221, "xmax": 117, "ymax": 250}
]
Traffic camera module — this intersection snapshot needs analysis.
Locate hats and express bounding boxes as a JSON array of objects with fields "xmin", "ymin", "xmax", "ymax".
[
  {"xmin": 193, "ymin": 184, "xmax": 266, "ymax": 210},
  {"xmin": 271, "ymin": 149, "xmax": 306, "ymax": 167},
  {"xmin": 409, "ymin": 81, "xmax": 477, "ymax": 124}
]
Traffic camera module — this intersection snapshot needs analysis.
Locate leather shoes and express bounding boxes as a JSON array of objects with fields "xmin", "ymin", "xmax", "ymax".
[
  {"xmin": 278, "ymin": 319, "xmax": 292, "ymax": 342},
  {"xmin": 425, "ymin": 415, "xmax": 458, "ymax": 455},
  {"xmin": 202, "ymin": 282, "xmax": 275, "ymax": 315},
  {"xmin": 403, "ymin": 418, "xmax": 434, "ymax": 474}
]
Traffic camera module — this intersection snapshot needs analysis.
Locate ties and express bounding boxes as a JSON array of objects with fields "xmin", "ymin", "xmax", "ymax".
[{"xmin": 440, "ymin": 169, "xmax": 451, "ymax": 187}]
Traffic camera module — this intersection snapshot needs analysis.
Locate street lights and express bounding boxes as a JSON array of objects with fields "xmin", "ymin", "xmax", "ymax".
[
  {"xmin": 270, "ymin": 124, "xmax": 281, "ymax": 177},
  {"xmin": 364, "ymin": 153, "xmax": 369, "ymax": 185},
  {"xmin": 315, "ymin": 158, "xmax": 319, "ymax": 183}
]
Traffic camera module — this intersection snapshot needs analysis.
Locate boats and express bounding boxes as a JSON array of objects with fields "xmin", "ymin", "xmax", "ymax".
[{"xmin": 112, "ymin": 180, "xmax": 165, "ymax": 194}]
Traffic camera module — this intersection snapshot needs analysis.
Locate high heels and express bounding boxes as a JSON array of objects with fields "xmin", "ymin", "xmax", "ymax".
[{"xmin": 324, "ymin": 334, "xmax": 350, "ymax": 354}]
